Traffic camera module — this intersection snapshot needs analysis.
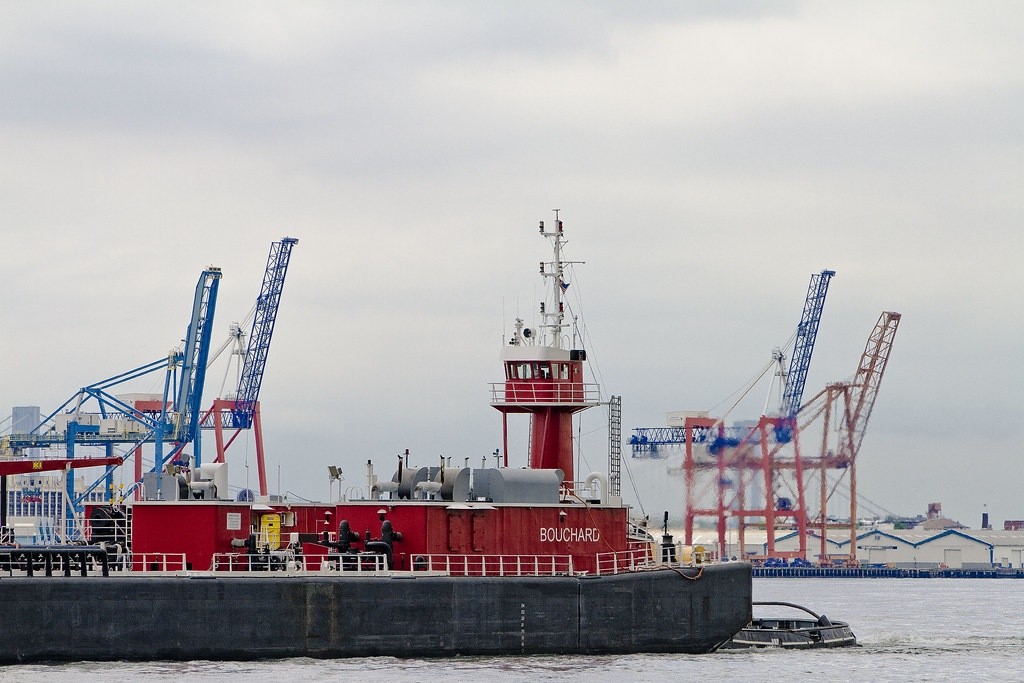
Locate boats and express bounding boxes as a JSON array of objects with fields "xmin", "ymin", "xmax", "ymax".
[
  {"xmin": 715, "ymin": 601, "xmax": 859, "ymax": 649},
  {"xmin": 0, "ymin": 209, "xmax": 759, "ymax": 662}
]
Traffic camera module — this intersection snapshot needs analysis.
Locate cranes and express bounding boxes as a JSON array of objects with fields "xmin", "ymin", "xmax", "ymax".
[
  {"xmin": 114, "ymin": 235, "xmax": 298, "ymax": 507},
  {"xmin": 0, "ymin": 265, "xmax": 226, "ymax": 544},
  {"xmin": 625, "ymin": 267, "xmax": 904, "ymax": 570}
]
